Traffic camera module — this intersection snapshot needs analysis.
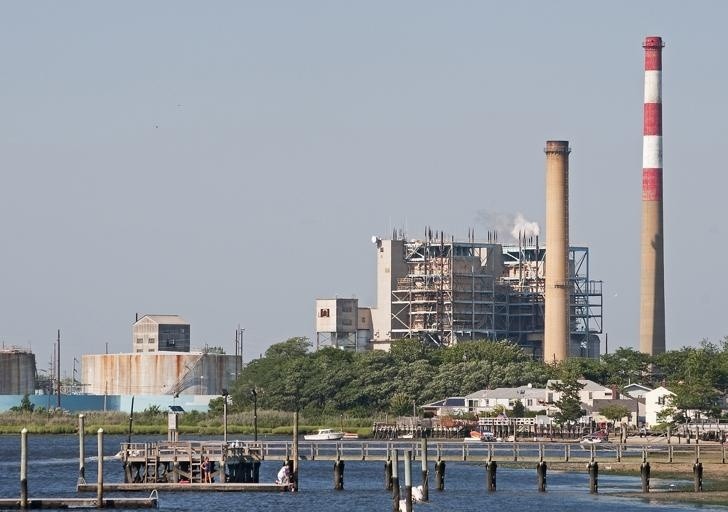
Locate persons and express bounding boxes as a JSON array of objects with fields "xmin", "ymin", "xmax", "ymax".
[
  {"xmin": 199, "ymin": 456, "xmax": 213, "ymax": 483},
  {"xmin": 275, "ymin": 463, "xmax": 292, "ymax": 485}
]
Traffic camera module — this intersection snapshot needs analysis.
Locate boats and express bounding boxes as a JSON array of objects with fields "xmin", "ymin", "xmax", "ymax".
[
  {"xmin": 579, "ymin": 431, "xmax": 619, "ymax": 451},
  {"xmin": 304, "ymin": 428, "xmax": 345, "ymax": 441}
]
